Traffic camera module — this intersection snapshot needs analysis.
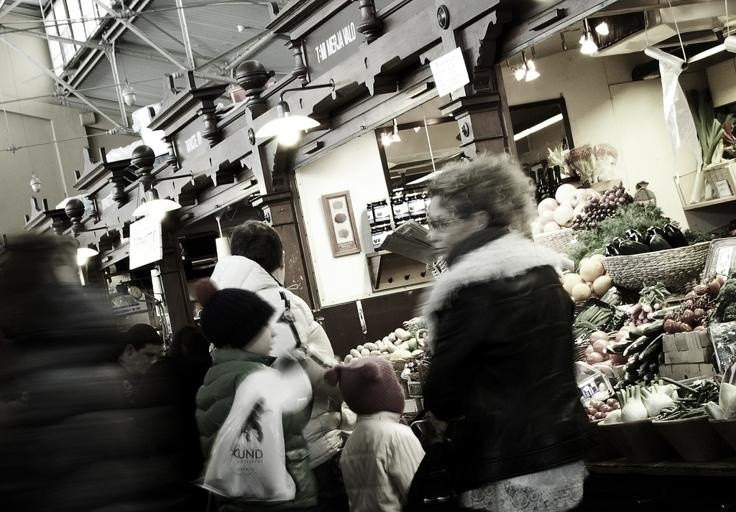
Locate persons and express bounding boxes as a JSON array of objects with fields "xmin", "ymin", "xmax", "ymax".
[
  {"xmin": 3, "ymin": 221, "xmax": 210, "ymax": 512},
  {"xmin": 413, "ymin": 150, "xmax": 597, "ymax": 511},
  {"xmin": 212, "ymin": 219, "xmax": 345, "ymax": 509},
  {"xmin": 325, "ymin": 356, "xmax": 428, "ymax": 511},
  {"xmin": 118, "ymin": 318, "xmax": 164, "ymax": 379},
  {"xmin": 189, "ymin": 276, "xmax": 319, "ymax": 510}
]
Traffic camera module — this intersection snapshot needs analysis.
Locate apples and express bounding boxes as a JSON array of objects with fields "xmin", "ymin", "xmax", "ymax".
[
  {"xmin": 531, "ymin": 184, "xmax": 600, "ymax": 235},
  {"xmin": 560, "ymin": 254, "xmax": 612, "ymax": 300}
]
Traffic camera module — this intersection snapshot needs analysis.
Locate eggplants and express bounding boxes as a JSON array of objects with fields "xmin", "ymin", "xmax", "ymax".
[{"xmin": 606, "ymin": 224, "xmax": 688, "ymax": 256}]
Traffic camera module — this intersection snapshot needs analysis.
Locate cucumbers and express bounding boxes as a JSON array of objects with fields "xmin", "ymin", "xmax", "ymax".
[{"xmin": 611, "ymin": 306, "xmax": 680, "ymax": 381}]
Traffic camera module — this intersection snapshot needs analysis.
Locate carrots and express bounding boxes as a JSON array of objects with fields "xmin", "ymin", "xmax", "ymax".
[
  {"xmin": 629, "ymin": 304, "xmax": 662, "ymax": 325},
  {"xmin": 664, "ymin": 275, "xmax": 724, "ymax": 332}
]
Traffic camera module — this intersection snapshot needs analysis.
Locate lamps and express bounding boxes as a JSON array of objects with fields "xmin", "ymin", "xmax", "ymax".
[
  {"xmin": 132, "ymin": 169, "xmax": 194, "ymax": 225},
  {"xmin": 254, "ymin": 79, "xmax": 336, "ymax": 149}
]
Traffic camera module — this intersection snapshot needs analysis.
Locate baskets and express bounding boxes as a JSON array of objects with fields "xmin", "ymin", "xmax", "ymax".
[
  {"xmin": 604, "ymin": 241, "xmax": 710, "ymax": 291},
  {"xmin": 572, "ymin": 344, "xmax": 589, "ymax": 362}
]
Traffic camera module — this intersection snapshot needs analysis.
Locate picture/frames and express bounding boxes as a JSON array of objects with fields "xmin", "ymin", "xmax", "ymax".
[{"xmin": 320, "ymin": 189, "xmax": 362, "ymax": 259}]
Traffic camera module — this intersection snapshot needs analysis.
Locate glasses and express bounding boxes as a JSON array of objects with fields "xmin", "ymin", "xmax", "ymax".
[{"xmin": 431, "ymin": 217, "xmax": 459, "ymax": 231}]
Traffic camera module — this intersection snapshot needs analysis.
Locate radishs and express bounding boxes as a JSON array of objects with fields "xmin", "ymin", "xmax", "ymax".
[{"xmin": 585, "ymin": 331, "xmax": 610, "ymax": 365}]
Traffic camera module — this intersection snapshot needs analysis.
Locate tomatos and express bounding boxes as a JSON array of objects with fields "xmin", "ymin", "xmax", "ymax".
[{"xmin": 587, "ymin": 399, "xmax": 620, "ymax": 420}]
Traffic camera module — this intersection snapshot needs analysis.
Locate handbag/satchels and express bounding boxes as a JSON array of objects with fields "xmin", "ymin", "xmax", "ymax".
[{"xmin": 400, "ymin": 432, "xmax": 473, "ymax": 511}]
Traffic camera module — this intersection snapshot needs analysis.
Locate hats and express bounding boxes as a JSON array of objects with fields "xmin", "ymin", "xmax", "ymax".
[
  {"xmin": 196, "ymin": 278, "xmax": 274, "ymax": 349},
  {"xmin": 325, "ymin": 357, "xmax": 404, "ymax": 413}
]
